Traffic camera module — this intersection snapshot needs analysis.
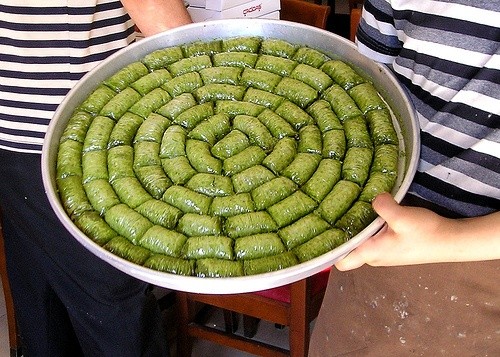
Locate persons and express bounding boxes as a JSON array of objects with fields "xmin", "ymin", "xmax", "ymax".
[
  {"xmin": 1, "ymin": 2, "xmax": 242, "ymax": 356},
  {"xmin": 313, "ymin": 2, "xmax": 500, "ymax": 273}
]
